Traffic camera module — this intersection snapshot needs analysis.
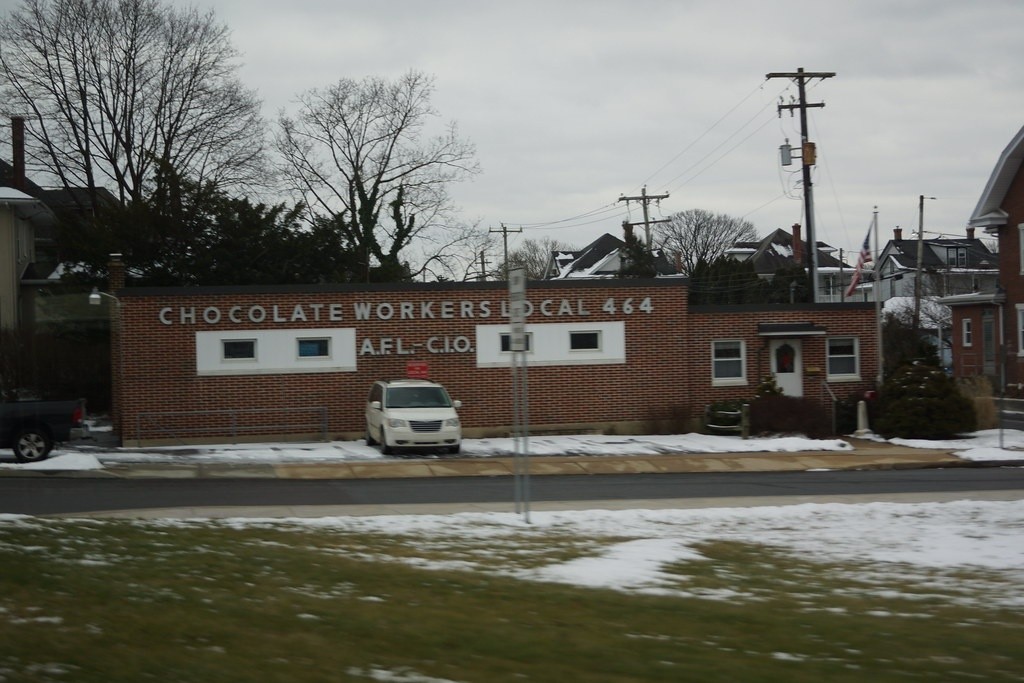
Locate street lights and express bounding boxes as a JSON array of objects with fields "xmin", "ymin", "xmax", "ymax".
[{"xmin": 912, "ymin": 196, "xmax": 938, "ymax": 360}]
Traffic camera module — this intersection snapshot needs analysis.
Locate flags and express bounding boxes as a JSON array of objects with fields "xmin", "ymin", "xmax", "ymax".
[{"xmin": 846, "ymin": 224, "xmax": 873, "ymax": 296}]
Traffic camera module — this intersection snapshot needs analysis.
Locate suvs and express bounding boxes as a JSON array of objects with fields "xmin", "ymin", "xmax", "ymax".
[{"xmin": 365, "ymin": 377, "xmax": 464, "ymax": 453}]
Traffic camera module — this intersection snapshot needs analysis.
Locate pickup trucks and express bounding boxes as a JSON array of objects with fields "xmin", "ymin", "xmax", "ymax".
[{"xmin": 0, "ymin": 398, "xmax": 86, "ymax": 461}]
